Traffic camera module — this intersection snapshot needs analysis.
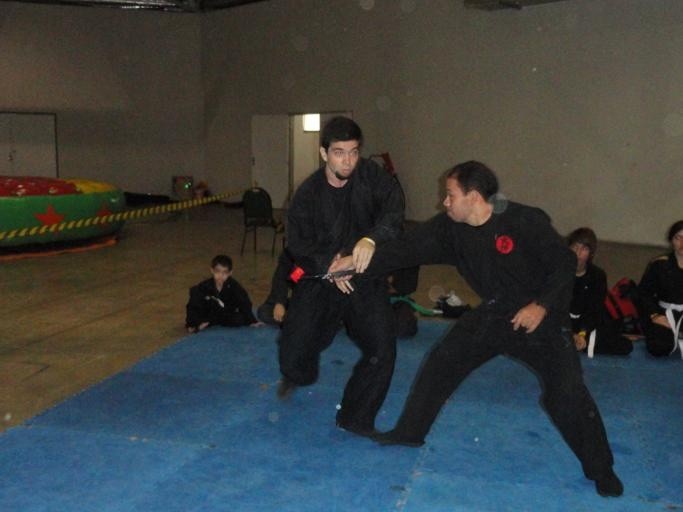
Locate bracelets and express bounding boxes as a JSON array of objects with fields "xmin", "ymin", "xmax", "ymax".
[{"xmin": 361, "ymin": 235, "xmax": 377, "ymax": 247}]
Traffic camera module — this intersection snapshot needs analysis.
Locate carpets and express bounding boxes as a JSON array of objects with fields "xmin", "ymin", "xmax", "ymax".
[{"xmin": 0, "ymin": 305, "xmax": 683, "ymax": 507}]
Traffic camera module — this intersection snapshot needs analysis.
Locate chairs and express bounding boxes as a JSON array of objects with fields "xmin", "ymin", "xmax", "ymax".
[{"xmin": 238, "ymin": 183, "xmax": 279, "ymax": 261}]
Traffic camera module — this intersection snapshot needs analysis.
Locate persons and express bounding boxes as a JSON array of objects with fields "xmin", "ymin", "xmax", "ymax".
[
  {"xmin": 274, "ymin": 114, "xmax": 408, "ymax": 437},
  {"xmin": 565, "ymin": 225, "xmax": 635, "ymax": 360},
  {"xmin": 327, "ymin": 159, "xmax": 626, "ymax": 499},
  {"xmin": 182, "ymin": 253, "xmax": 269, "ymax": 334},
  {"xmin": 255, "ymin": 246, "xmax": 301, "ymax": 329},
  {"xmin": 386, "ymin": 221, "xmax": 422, "ymax": 340},
  {"xmin": 633, "ymin": 219, "xmax": 683, "ymax": 360}
]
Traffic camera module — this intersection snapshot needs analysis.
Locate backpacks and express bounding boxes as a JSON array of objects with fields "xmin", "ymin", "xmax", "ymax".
[{"xmin": 606, "ymin": 277, "xmax": 648, "ymax": 341}]
